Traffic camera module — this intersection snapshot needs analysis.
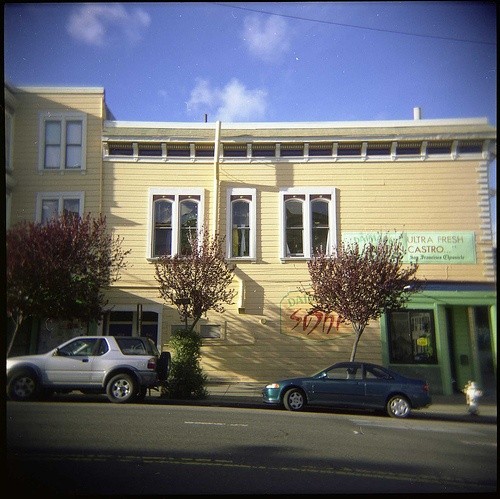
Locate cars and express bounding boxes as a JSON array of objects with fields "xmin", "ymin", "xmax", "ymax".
[{"xmin": 263, "ymin": 361, "xmax": 433, "ymax": 419}]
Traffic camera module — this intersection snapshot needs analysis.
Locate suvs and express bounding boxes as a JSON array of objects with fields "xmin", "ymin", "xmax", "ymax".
[{"xmin": 5, "ymin": 336, "xmax": 171, "ymax": 405}]
